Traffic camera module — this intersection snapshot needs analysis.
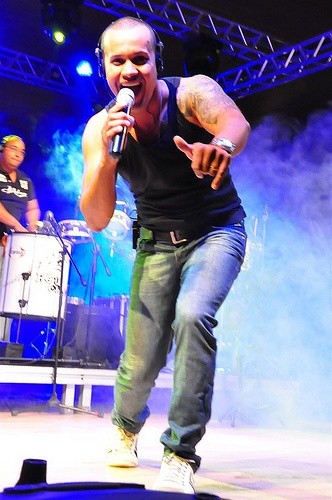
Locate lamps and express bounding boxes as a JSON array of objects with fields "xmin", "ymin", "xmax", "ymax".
[{"xmin": 39, "ymin": 0, "xmax": 79, "ymax": 45}]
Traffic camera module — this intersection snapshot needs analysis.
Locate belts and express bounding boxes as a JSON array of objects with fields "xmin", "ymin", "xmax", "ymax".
[{"xmin": 139, "ymin": 225, "xmax": 216, "ymax": 245}]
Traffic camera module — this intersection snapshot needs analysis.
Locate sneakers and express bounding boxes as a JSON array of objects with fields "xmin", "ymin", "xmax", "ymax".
[
  {"xmin": 104, "ymin": 423, "xmax": 138, "ymax": 466},
  {"xmin": 150, "ymin": 445, "xmax": 196, "ymax": 494}
]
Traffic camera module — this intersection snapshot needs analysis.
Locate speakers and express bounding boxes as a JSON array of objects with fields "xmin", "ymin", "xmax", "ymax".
[{"xmin": 62, "ymin": 303, "xmax": 110, "ymax": 362}]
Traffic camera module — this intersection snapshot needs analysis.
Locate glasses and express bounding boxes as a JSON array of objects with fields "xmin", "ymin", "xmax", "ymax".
[{"xmin": 4, "ymin": 143, "xmax": 27, "ymax": 156}]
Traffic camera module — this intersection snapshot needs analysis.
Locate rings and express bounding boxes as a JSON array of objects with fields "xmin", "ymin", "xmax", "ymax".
[{"xmin": 210, "ymin": 165, "xmax": 218, "ymax": 171}]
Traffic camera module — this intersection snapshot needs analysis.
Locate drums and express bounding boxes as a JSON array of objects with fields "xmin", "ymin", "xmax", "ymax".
[
  {"xmin": 100, "ymin": 210, "xmax": 131, "ymax": 241},
  {"xmin": 0, "ymin": 231, "xmax": 73, "ymax": 321},
  {"xmin": 58, "ymin": 221, "xmax": 92, "ymax": 243}
]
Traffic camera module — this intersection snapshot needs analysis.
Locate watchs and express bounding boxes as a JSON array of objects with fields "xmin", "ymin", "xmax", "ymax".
[{"xmin": 211, "ymin": 137, "xmax": 236, "ymax": 155}]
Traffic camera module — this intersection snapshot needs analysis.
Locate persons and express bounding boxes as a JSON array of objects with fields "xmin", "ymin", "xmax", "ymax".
[
  {"xmin": 80, "ymin": 17, "xmax": 250, "ymax": 492},
  {"xmin": 0, "ymin": 135, "xmax": 41, "ymax": 343}
]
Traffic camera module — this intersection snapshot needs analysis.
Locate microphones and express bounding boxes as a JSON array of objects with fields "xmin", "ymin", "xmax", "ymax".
[
  {"xmin": 111, "ymin": 88, "xmax": 135, "ymax": 153},
  {"xmin": 45, "ymin": 210, "xmax": 63, "ymax": 232}
]
